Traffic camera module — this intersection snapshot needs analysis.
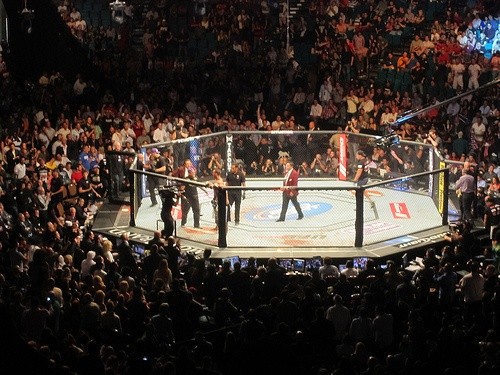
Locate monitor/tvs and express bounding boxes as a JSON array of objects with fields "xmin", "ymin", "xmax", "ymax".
[
  {"xmin": 279, "ymin": 257, "xmax": 323, "ymax": 273},
  {"xmin": 339, "ymin": 265, "xmax": 346, "ymax": 270},
  {"xmin": 402, "ymin": 253, "xmax": 408, "ymax": 267},
  {"xmin": 134, "ymin": 244, "xmax": 145, "ymax": 253},
  {"xmin": 222, "ymin": 255, "xmax": 257, "ymax": 270},
  {"xmin": 353, "ymin": 257, "xmax": 367, "ymax": 268}
]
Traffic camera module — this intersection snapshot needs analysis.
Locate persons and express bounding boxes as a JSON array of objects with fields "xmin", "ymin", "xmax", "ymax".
[
  {"xmin": 178, "ymin": 168, "xmax": 209, "ymax": 229},
  {"xmin": 160, "ymin": 189, "xmax": 182, "ymax": 240},
  {"xmin": 142, "ymin": 148, "xmax": 167, "ymax": 208},
  {"xmin": 168, "ymin": 159, "xmax": 204, "ymax": 217},
  {"xmin": 208, "ymin": 167, "xmax": 230, "ymax": 232},
  {"xmin": 353, "ymin": 150, "xmax": 377, "ymax": 211},
  {"xmin": 0, "ymin": 0, "xmax": 500, "ymax": 375},
  {"xmin": 207, "ymin": 150, "xmax": 224, "ymax": 182},
  {"xmin": 275, "ymin": 160, "xmax": 304, "ymax": 223},
  {"xmin": 454, "ymin": 168, "xmax": 476, "ymax": 230},
  {"xmin": 225, "ymin": 163, "xmax": 246, "ymax": 226}
]
